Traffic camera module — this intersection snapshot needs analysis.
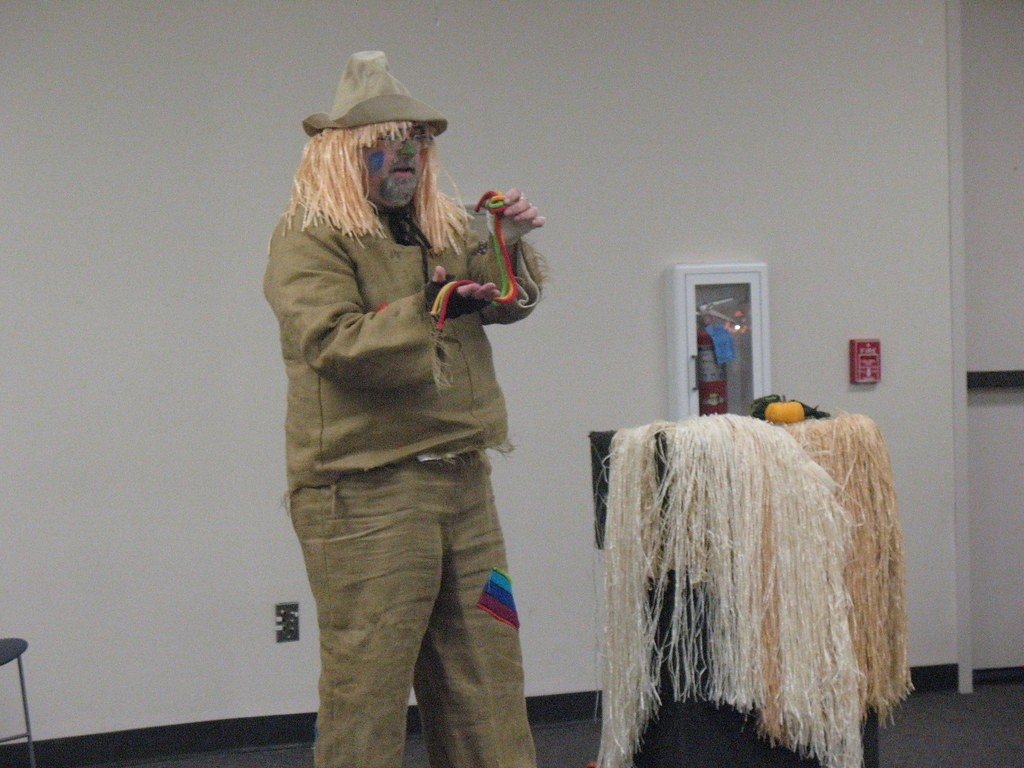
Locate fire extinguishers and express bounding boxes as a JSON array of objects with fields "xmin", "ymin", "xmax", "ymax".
[{"xmin": 697, "ymin": 298, "xmax": 735, "ymax": 415}]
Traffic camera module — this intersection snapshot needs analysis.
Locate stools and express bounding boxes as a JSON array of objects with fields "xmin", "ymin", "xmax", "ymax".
[{"xmin": 0, "ymin": 638, "xmax": 36, "ymax": 768}]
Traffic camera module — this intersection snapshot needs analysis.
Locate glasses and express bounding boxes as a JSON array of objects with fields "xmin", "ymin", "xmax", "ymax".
[{"xmin": 377, "ymin": 133, "xmax": 435, "ymax": 151}]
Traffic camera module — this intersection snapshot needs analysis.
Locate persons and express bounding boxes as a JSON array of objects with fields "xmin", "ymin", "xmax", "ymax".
[{"xmin": 262, "ymin": 50, "xmax": 548, "ymax": 768}]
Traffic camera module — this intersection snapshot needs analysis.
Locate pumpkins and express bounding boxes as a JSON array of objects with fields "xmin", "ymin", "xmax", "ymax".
[{"xmin": 764, "ymin": 394, "xmax": 805, "ymax": 424}]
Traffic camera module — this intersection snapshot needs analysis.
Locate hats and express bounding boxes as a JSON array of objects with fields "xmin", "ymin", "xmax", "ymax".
[{"xmin": 302, "ymin": 50, "xmax": 447, "ymax": 137}]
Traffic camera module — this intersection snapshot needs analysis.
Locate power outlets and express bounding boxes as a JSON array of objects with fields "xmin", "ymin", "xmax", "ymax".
[{"xmin": 274, "ymin": 601, "xmax": 301, "ymax": 643}]
